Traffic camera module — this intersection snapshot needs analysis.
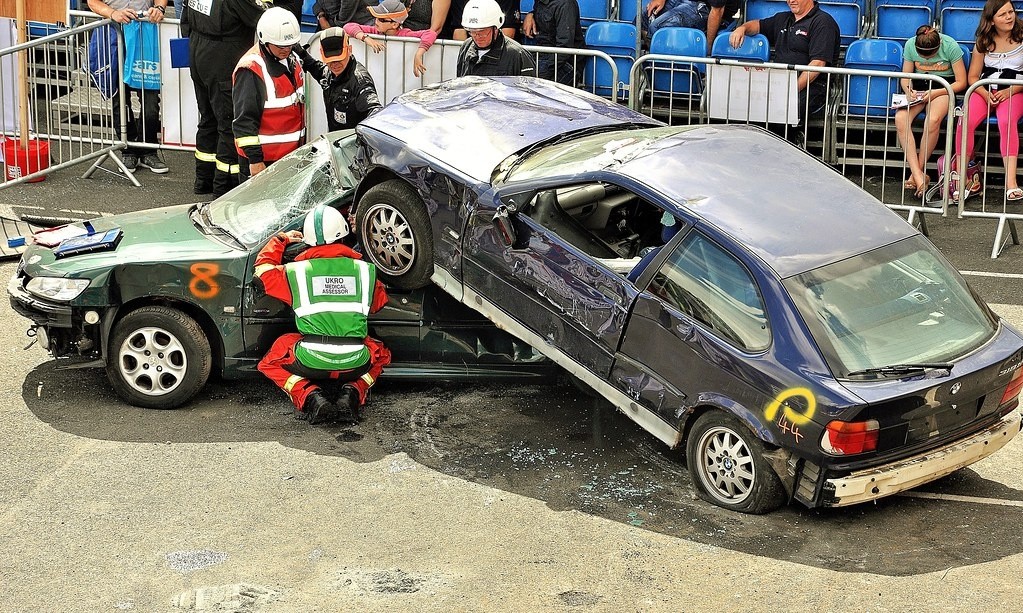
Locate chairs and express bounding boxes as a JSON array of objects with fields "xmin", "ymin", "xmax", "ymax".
[
  {"xmin": 26, "ymin": 0, "xmax": 84, "ymax": 78},
  {"xmin": 298, "ymin": 0, "xmax": 1023, "ymax": 181}
]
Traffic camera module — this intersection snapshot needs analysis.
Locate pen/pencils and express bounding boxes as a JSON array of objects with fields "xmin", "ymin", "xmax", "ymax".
[{"xmin": 907, "ymin": 85, "xmax": 918, "ymax": 100}]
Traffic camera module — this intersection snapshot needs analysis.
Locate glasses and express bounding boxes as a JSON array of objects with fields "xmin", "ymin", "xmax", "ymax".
[
  {"xmin": 468, "ymin": 27, "xmax": 493, "ymax": 38},
  {"xmin": 378, "ymin": 18, "xmax": 395, "ymax": 22}
]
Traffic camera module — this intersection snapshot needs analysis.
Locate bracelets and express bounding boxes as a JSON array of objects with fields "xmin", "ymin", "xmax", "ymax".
[{"xmin": 110, "ymin": 10, "xmax": 115, "ymax": 19}]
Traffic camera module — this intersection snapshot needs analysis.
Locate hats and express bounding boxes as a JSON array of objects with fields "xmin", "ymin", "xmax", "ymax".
[
  {"xmin": 367, "ymin": 0, "xmax": 408, "ymax": 24},
  {"xmin": 319, "ymin": 27, "xmax": 348, "ymax": 64}
]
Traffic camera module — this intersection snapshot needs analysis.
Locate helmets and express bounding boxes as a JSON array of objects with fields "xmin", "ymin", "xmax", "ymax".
[
  {"xmin": 303, "ymin": 206, "xmax": 349, "ymax": 246},
  {"xmin": 461, "ymin": 0, "xmax": 505, "ymax": 30},
  {"xmin": 256, "ymin": 7, "xmax": 301, "ymax": 46}
]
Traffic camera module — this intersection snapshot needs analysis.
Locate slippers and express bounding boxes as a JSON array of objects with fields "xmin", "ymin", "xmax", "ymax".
[
  {"xmin": 1006, "ymin": 188, "xmax": 1023, "ymax": 200},
  {"xmin": 952, "ymin": 182, "xmax": 974, "ymax": 202}
]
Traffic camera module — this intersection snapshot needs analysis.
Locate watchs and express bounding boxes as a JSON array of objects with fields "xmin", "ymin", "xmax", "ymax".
[
  {"xmin": 316, "ymin": 14, "xmax": 325, "ymax": 21},
  {"xmin": 155, "ymin": 5, "xmax": 165, "ymax": 15},
  {"xmin": 361, "ymin": 34, "xmax": 368, "ymax": 42}
]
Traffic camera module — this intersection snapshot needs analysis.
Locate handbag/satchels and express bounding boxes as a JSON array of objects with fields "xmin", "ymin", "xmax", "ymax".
[
  {"xmin": 926, "ymin": 154, "xmax": 983, "ymax": 207},
  {"xmin": 122, "ymin": 12, "xmax": 161, "ymax": 90}
]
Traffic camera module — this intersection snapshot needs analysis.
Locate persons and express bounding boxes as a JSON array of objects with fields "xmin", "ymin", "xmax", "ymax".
[
  {"xmin": 253, "ymin": 202, "xmax": 391, "ymax": 424},
  {"xmin": 729, "ymin": 0, "xmax": 841, "ymax": 145},
  {"xmin": 173, "ymin": 0, "xmax": 304, "ymax": 198},
  {"xmin": 294, "ymin": 27, "xmax": 382, "ymax": 133},
  {"xmin": 895, "ymin": 25, "xmax": 967, "ymax": 198},
  {"xmin": 231, "ymin": 6, "xmax": 307, "ymax": 186},
  {"xmin": 954, "ymin": 0, "xmax": 1023, "ymax": 206},
  {"xmin": 521, "ymin": 0, "xmax": 585, "ymax": 87},
  {"xmin": 632, "ymin": 0, "xmax": 740, "ymax": 57},
  {"xmin": 87, "ymin": 0, "xmax": 168, "ymax": 173},
  {"xmin": 311, "ymin": 0, "xmax": 525, "ymax": 77},
  {"xmin": 456, "ymin": 0, "xmax": 536, "ymax": 77}
]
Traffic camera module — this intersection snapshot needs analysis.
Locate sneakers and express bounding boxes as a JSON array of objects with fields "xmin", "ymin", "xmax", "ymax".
[
  {"xmin": 336, "ymin": 388, "xmax": 357, "ymax": 422},
  {"xmin": 138, "ymin": 155, "xmax": 168, "ymax": 173},
  {"xmin": 119, "ymin": 157, "xmax": 137, "ymax": 173},
  {"xmin": 307, "ymin": 394, "xmax": 331, "ymax": 425}
]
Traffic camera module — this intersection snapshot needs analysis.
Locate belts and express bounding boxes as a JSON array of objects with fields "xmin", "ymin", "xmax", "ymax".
[{"xmin": 303, "ymin": 334, "xmax": 363, "ymax": 345}]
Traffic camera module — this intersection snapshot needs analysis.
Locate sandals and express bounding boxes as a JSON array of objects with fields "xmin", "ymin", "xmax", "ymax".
[
  {"xmin": 904, "ymin": 180, "xmax": 916, "ymax": 189},
  {"xmin": 913, "ymin": 175, "xmax": 930, "ymax": 198}
]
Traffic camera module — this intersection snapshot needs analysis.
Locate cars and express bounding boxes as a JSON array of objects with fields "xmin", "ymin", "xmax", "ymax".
[
  {"xmin": 6, "ymin": 123, "xmax": 577, "ymax": 410},
  {"xmin": 354, "ymin": 74, "xmax": 1023, "ymax": 516}
]
